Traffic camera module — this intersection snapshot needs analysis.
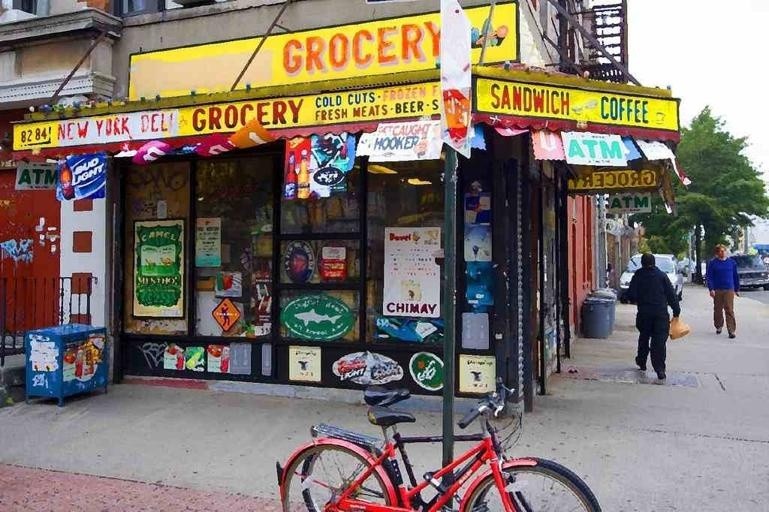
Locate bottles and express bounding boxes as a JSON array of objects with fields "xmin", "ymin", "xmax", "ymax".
[
  {"xmin": 283, "ymin": 151, "xmax": 297, "ymax": 201},
  {"xmin": 297, "ymin": 148, "xmax": 311, "ymax": 199},
  {"xmin": 59, "ymin": 162, "xmax": 76, "ymax": 200}
]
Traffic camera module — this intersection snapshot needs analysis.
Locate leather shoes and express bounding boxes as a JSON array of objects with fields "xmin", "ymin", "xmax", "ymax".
[
  {"xmin": 635, "ymin": 356, "xmax": 647, "ymax": 370},
  {"xmin": 658, "ymin": 369, "xmax": 667, "ymax": 380}
]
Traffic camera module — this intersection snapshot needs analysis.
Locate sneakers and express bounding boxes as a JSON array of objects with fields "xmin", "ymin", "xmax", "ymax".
[
  {"xmin": 729, "ymin": 332, "xmax": 736, "ymax": 338},
  {"xmin": 716, "ymin": 327, "xmax": 723, "ymax": 334}
]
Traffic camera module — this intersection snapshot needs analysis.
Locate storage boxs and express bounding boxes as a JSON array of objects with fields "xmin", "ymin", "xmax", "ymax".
[{"xmin": 24, "ymin": 322, "xmax": 108, "ymax": 407}]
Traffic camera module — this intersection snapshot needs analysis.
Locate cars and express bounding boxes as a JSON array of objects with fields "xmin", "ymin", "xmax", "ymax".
[
  {"xmin": 619, "ymin": 253, "xmax": 683, "ymax": 304},
  {"xmin": 729, "ymin": 254, "xmax": 768, "ymax": 291}
]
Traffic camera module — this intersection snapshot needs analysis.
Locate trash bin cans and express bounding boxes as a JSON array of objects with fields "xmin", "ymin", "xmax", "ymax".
[{"xmin": 580, "ymin": 288, "xmax": 617, "ymax": 338}]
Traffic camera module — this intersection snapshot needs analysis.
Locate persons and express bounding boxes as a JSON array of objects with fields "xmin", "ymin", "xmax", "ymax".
[
  {"xmin": 706, "ymin": 242, "xmax": 742, "ymax": 340},
  {"xmin": 628, "ymin": 252, "xmax": 680, "ymax": 380}
]
